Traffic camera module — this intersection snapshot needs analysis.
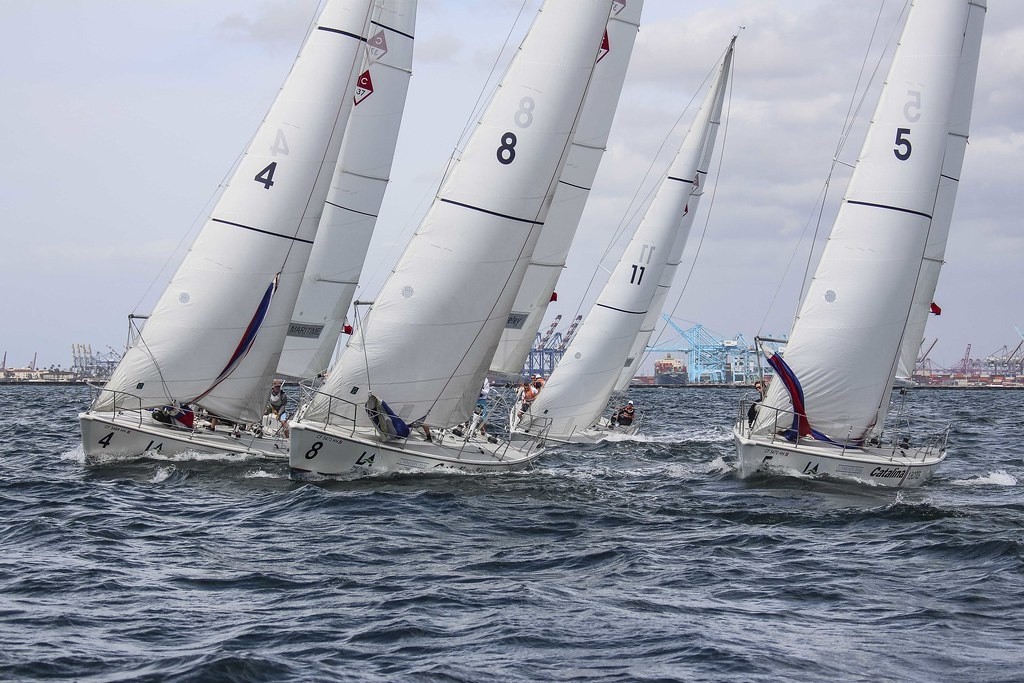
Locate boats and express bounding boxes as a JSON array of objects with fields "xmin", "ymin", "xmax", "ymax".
[{"xmin": 652, "ymin": 353, "xmax": 689, "ymax": 385}]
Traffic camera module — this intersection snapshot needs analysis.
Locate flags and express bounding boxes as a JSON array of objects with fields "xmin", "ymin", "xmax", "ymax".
[
  {"xmin": 928, "ymin": 301, "xmax": 941, "ymax": 315},
  {"xmin": 343, "ymin": 316, "xmax": 354, "ymax": 335},
  {"xmin": 549, "ymin": 292, "xmax": 557, "ymax": 302}
]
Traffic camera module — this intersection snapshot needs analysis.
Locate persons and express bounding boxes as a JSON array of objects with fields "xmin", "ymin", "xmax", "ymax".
[
  {"xmin": 255, "ymin": 379, "xmax": 290, "ymax": 439},
  {"xmin": 477, "ymin": 377, "xmax": 489, "ymax": 420},
  {"xmin": 423, "ymin": 426, "xmax": 431, "ymax": 442},
  {"xmin": 608, "ymin": 401, "xmax": 634, "ymax": 430},
  {"xmin": 518, "ymin": 373, "xmax": 549, "ymax": 420},
  {"xmin": 203, "ymin": 417, "xmax": 240, "ymax": 437},
  {"xmin": 755, "ymin": 381, "xmax": 766, "ymax": 393}
]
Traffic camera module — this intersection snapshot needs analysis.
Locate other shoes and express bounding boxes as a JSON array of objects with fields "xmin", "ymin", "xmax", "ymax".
[
  {"xmin": 423, "ymin": 439, "xmax": 432, "ymax": 443},
  {"xmin": 203, "ymin": 424, "xmax": 215, "ymax": 432}
]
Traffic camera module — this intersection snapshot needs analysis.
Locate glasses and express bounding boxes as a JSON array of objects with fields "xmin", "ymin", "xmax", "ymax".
[
  {"xmin": 531, "ymin": 378, "xmax": 535, "ymax": 379},
  {"xmin": 629, "ymin": 403, "xmax": 633, "ymax": 406},
  {"xmin": 756, "ymin": 386, "xmax": 761, "ymax": 390}
]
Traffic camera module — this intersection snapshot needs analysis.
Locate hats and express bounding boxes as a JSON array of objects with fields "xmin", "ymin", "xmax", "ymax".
[{"xmin": 627, "ymin": 400, "xmax": 633, "ymax": 405}]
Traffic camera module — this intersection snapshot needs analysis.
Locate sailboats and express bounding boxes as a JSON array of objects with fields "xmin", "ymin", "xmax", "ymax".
[
  {"xmin": 509, "ymin": 34, "xmax": 738, "ymax": 452},
  {"xmin": 731, "ymin": 0, "xmax": 988, "ymax": 492},
  {"xmin": 77, "ymin": 2, "xmax": 419, "ymax": 462},
  {"xmin": 287, "ymin": 0, "xmax": 644, "ymax": 479}
]
